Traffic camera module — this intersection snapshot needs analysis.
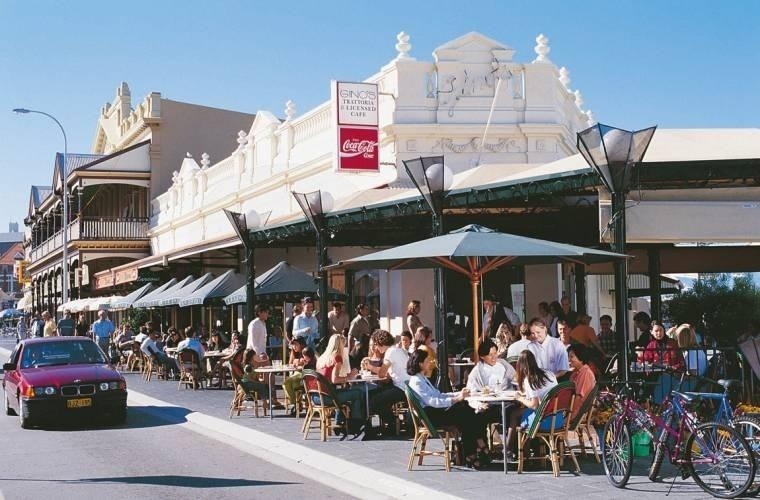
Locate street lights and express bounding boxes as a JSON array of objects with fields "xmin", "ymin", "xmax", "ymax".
[
  {"xmin": 221, "ymin": 207, "xmax": 272, "ymax": 345},
  {"xmin": 574, "ymin": 122, "xmax": 659, "ymax": 399},
  {"xmin": 399, "ymin": 157, "xmax": 456, "ymax": 393},
  {"xmin": 13, "ymin": 109, "xmax": 71, "ymax": 336},
  {"xmin": 291, "ymin": 189, "xmax": 334, "ymax": 357}
]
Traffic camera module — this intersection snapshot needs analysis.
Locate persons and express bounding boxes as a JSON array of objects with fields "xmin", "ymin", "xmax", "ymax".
[{"xmin": 13, "ymin": 293, "xmax": 759, "ymax": 473}]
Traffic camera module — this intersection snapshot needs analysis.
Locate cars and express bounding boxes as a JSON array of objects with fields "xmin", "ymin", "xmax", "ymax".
[{"xmin": 1, "ymin": 336, "xmax": 125, "ymax": 432}]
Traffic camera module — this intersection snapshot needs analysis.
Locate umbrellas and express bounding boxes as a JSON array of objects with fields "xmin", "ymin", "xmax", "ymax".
[
  {"xmin": 317, "ymin": 221, "xmax": 637, "ymax": 365},
  {"xmin": 57, "ymin": 271, "xmax": 250, "ymax": 336},
  {"xmin": 0, "ymin": 308, "xmax": 24, "ymax": 331},
  {"xmin": 222, "ymin": 259, "xmax": 346, "ymax": 400}
]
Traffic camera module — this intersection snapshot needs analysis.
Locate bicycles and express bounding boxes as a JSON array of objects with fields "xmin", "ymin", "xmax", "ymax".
[
  {"xmin": 648, "ymin": 365, "xmax": 759, "ymax": 493},
  {"xmin": 600, "ymin": 386, "xmax": 755, "ymax": 499}
]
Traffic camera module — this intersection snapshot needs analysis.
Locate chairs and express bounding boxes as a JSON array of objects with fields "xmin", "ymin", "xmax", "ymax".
[{"xmin": 114, "ymin": 340, "xmax": 206, "ymax": 392}]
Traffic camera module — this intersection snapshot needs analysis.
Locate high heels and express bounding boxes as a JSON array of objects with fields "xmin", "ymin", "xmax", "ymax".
[
  {"xmin": 465, "ymin": 457, "xmax": 480, "ymax": 470},
  {"xmin": 476, "ymin": 445, "xmax": 492, "ymax": 459}
]
